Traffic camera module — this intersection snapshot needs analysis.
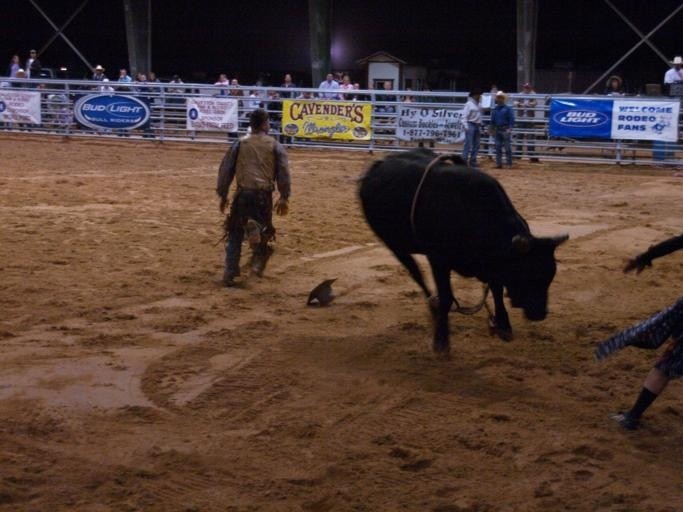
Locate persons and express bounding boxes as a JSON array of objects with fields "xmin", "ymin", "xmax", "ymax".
[
  {"xmin": 8, "ymin": 49, "xmax": 80, "ymax": 132},
  {"xmin": 417, "ymin": 83, "xmax": 438, "ymax": 148},
  {"xmin": 603, "ymin": 75, "xmax": 625, "ymax": 97},
  {"xmin": 596, "ymin": 231, "xmax": 683, "ymax": 430},
  {"xmin": 218, "ymin": 109, "xmax": 290, "ymax": 286},
  {"xmin": 460, "ymin": 86, "xmax": 485, "ymax": 167},
  {"xmin": 400, "ymin": 88, "xmax": 416, "ymax": 145},
  {"xmin": 482, "ymin": 85, "xmax": 498, "ymax": 124},
  {"xmin": 77, "ymin": 64, "xmax": 186, "ymax": 138},
  {"xmin": 664, "ymin": 56, "xmax": 683, "ymax": 86},
  {"xmin": 486, "ymin": 90, "xmax": 514, "ymax": 169},
  {"xmin": 317, "ymin": 73, "xmax": 368, "ymax": 142},
  {"xmin": 513, "ymin": 83, "xmax": 537, "ymax": 159},
  {"xmin": 213, "ymin": 73, "xmax": 315, "ymax": 144},
  {"xmin": 375, "ymin": 80, "xmax": 397, "ymax": 135}
]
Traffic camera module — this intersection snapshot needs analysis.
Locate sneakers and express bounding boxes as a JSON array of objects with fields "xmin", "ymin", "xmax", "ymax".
[{"xmin": 609, "ymin": 410, "xmax": 636, "ymax": 430}]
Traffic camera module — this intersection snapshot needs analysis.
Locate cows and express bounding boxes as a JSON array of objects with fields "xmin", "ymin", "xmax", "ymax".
[{"xmin": 356, "ymin": 146, "xmax": 570, "ymax": 361}]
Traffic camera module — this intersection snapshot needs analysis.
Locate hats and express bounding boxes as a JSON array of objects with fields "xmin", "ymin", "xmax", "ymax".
[
  {"xmin": 607, "ymin": 76, "xmax": 623, "ymax": 88},
  {"xmin": 95, "ymin": 65, "xmax": 105, "ymax": 73},
  {"xmin": 306, "ymin": 277, "xmax": 337, "ymax": 307},
  {"xmin": 469, "ymin": 88, "xmax": 484, "ymax": 97},
  {"xmin": 495, "ymin": 90, "xmax": 506, "ymax": 100},
  {"xmin": 670, "ymin": 56, "xmax": 683, "ymax": 65}
]
